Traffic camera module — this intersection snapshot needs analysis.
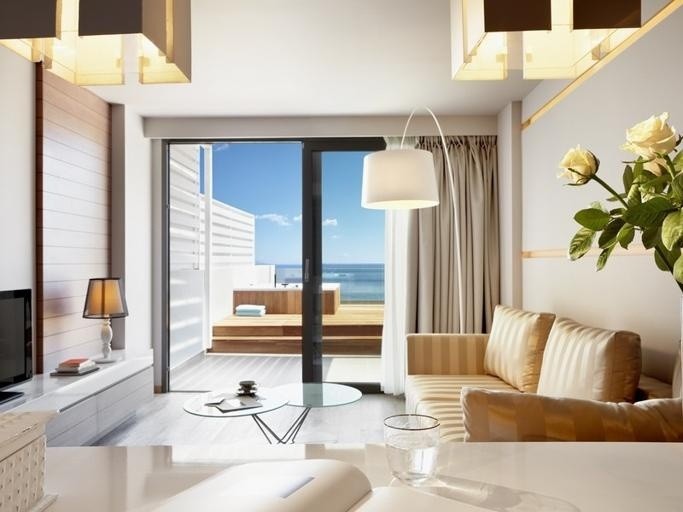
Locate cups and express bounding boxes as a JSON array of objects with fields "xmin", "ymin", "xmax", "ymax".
[{"xmin": 383, "ymin": 412, "xmax": 441, "ymax": 486}]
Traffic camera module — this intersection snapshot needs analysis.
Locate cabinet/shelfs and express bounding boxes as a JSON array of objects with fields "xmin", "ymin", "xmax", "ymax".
[{"xmin": 0, "ymin": 356, "xmax": 156, "ymax": 447}]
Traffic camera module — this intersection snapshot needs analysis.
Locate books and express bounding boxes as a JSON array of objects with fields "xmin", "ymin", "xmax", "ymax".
[
  {"xmin": 204, "ymin": 390, "xmax": 227, "ymax": 407},
  {"xmin": 53, "ymin": 360, "xmax": 96, "ymax": 372},
  {"xmin": 215, "ymin": 395, "xmax": 262, "ymax": 413},
  {"xmin": 58, "ymin": 357, "xmax": 90, "ymax": 367},
  {"xmin": 49, "ymin": 366, "xmax": 100, "ymax": 377}
]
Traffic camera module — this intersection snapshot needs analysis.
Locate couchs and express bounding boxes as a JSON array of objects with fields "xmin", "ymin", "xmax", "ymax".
[{"xmin": 403, "ymin": 303, "xmax": 643, "ymax": 442}]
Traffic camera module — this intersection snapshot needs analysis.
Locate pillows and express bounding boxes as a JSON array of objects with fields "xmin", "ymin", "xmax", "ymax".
[
  {"xmin": 483, "ymin": 305, "xmax": 642, "ymax": 406},
  {"xmin": 460, "ymin": 384, "xmax": 682, "ymax": 443}
]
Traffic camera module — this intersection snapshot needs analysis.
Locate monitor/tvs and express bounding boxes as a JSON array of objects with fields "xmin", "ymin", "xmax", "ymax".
[{"xmin": 0, "ymin": 288, "xmax": 33, "ymax": 404}]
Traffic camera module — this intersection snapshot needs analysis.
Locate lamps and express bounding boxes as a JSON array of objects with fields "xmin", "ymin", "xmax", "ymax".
[
  {"xmin": 82, "ymin": 276, "xmax": 131, "ymax": 364},
  {"xmin": 450, "ymin": 1, "xmax": 641, "ymax": 82},
  {"xmin": 362, "ymin": 108, "xmax": 467, "ymax": 334},
  {"xmin": 1, "ymin": 1, "xmax": 192, "ymax": 87}
]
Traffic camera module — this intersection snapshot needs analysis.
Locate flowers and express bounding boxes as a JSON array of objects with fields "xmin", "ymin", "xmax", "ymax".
[{"xmin": 555, "ymin": 111, "xmax": 683, "ymax": 291}]
